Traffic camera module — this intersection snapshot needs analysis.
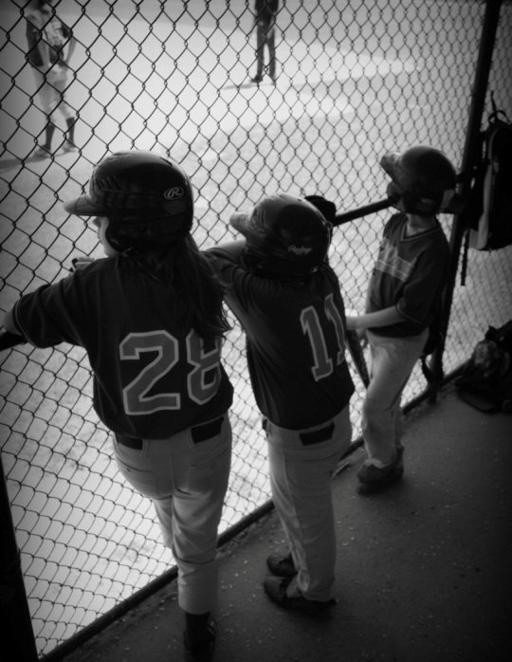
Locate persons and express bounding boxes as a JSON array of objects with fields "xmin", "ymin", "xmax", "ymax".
[
  {"xmin": 343, "ymin": 142, "xmax": 464, "ymax": 502},
  {"xmin": 197, "ymin": 191, "xmax": 361, "ymax": 628},
  {"xmin": 20, "ymin": 0, "xmax": 78, "ymax": 159},
  {"xmin": 0, "ymin": 147, "xmax": 239, "ymax": 660},
  {"xmin": 250, "ymin": 0, "xmax": 280, "ymax": 83}
]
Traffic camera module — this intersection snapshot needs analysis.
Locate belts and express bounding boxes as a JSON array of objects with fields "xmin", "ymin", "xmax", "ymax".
[
  {"xmin": 115, "ymin": 417, "xmax": 224, "ymax": 452},
  {"xmin": 298, "ymin": 421, "xmax": 336, "ymax": 444}
]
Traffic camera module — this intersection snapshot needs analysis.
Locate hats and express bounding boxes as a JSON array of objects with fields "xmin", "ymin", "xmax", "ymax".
[
  {"xmin": 63, "ymin": 150, "xmax": 196, "ymax": 254},
  {"xmin": 229, "ymin": 191, "xmax": 334, "ymax": 266},
  {"xmin": 378, "ymin": 145, "xmax": 458, "ymax": 217}
]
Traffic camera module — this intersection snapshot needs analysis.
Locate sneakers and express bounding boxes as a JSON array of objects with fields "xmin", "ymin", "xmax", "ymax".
[
  {"xmin": 39, "ymin": 144, "xmax": 51, "ymax": 152},
  {"xmin": 63, "ymin": 138, "xmax": 75, "ymax": 149},
  {"xmin": 182, "ymin": 614, "xmax": 217, "ymax": 660},
  {"xmin": 263, "ymin": 577, "xmax": 329, "ymax": 617},
  {"xmin": 356, "ymin": 447, "xmax": 407, "ymax": 498},
  {"xmin": 266, "ymin": 552, "xmax": 298, "ymax": 578}
]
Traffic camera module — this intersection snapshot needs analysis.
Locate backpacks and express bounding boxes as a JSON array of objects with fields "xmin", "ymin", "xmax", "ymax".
[
  {"xmin": 460, "ymin": 109, "xmax": 511, "ymax": 251},
  {"xmin": 454, "ymin": 320, "xmax": 511, "ymax": 418}
]
